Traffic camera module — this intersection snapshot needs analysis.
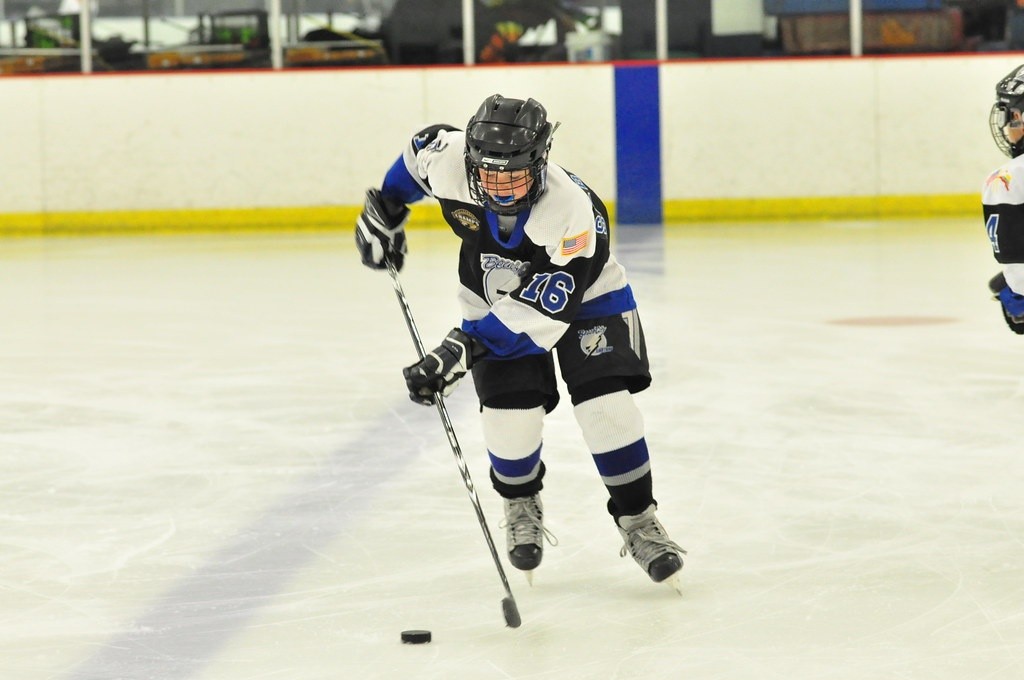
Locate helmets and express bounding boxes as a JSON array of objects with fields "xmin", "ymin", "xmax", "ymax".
[
  {"xmin": 463, "ymin": 94, "xmax": 561, "ymax": 216},
  {"xmin": 989, "ymin": 64, "xmax": 1024, "ymax": 157}
]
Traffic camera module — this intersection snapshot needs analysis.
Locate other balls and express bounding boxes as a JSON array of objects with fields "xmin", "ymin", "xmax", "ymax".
[{"xmin": 400, "ymin": 630, "xmax": 433, "ymax": 644}]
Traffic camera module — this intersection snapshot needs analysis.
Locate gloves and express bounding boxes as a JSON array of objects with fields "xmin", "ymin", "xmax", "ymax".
[
  {"xmin": 355, "ymin": 189, "xmax": 410, "ymax": 270},
  {"xmin": 403, "ymin": 328, "xmax": 488, "ymax": 406},
  {"xmin": 995, "ymin": 287, "xmax": 1024, "ymax": 335}
]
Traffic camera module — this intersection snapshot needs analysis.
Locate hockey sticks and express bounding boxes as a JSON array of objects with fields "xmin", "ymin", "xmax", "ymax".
[{"xmin": 363, "ymin": 186, "xmax": 522, "ymax": 630}]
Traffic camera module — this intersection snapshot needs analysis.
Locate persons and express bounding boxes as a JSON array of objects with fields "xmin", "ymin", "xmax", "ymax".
[
  {"xmin": 354, "ymin": 94, "xmax": 684, "ymax": 598},
  {"xmin": 981, "ymin": 63, "xmax": 1024, "ymax": 335}
]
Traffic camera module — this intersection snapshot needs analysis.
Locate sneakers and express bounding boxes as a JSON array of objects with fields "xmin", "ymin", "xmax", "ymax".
[
  {"xmin": 499, "ymin": 493, "xmax": 558, "ymax": 586},
  {"xmin": 607, "ymin": 496, "xmax": 687, "ymax": 599}
]
